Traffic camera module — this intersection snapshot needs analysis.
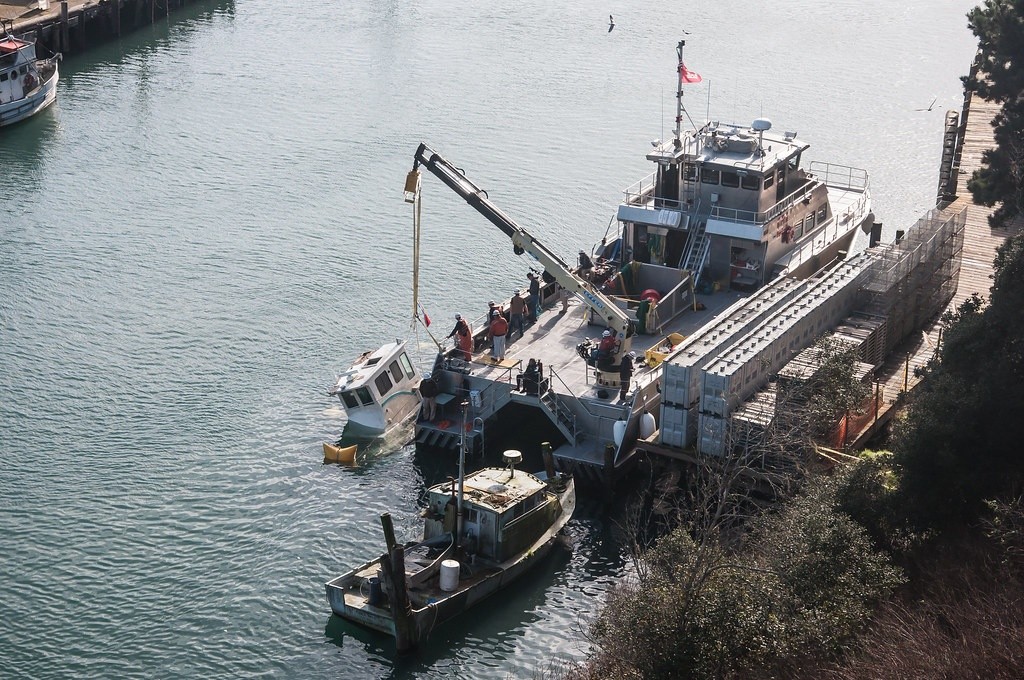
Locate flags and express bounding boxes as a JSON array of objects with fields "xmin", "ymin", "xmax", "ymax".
[
  {"xmin": 679, "ymin": 64, "xmax": 702, "ymax": 86},
  {"xmin": 417, "ymin": 300, "xmax": 431, "ymax": 327}
]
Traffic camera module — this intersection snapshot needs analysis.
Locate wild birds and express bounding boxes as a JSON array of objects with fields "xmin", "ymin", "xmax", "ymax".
[
  {"xmin": 913, "ymin": 97, "xmax": 937, "ymax": 112},
  {"xmin": 682, "ymin": 29, "xmax": 693, "ymax": 36},
  {"xmin": 607, "ymin": 14, "xmax": 616, "ymax": 33}
]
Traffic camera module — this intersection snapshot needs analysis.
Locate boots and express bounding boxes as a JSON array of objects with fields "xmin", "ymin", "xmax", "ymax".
[{"xmin": 620, "ymin": 391, "xmax": 626, "ymax": 400}]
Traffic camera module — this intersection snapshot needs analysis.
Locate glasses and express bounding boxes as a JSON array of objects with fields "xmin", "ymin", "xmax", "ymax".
[{"xmin": 528, "ymin": 276, "xmax": 531, "ymax": 278}]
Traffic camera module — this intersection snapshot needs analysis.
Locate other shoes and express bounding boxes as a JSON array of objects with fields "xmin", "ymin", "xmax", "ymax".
[
  {"xmin": 532, "ymin": 319, "xmax": 537, "ymax": 322},
  {"xmin": 561, "ymin": 310, "xmax": 567, "ymax": 313},
  {"xmin": 512, "ymin": 388, "xmax": 520, "ymax": 391},
  {"xmin": 500, "ymin": 359, "xmax": 504, "ymax": 361},
  {"xmin": 491, "ymin": 357, "xmax": 498, "ymax": 362},
  {"xmin": 464, "ymin": 358, "xmax": 472, "ymax": 361},
  {"xmin": 505, "ymin": 335, "xmax": 511, "ymax": 338},
  {"xmin": 520, "ymin": 334, "xmax": 524, "ymax": 337},
  {"xmin": 520, "ymin": 389, "xmax": 526, "ymax": 393}
]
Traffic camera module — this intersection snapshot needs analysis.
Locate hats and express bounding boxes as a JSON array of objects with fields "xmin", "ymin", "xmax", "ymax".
[{"xmin": 526, "ymin": 272, "xmax": 534, "ymax": 278}]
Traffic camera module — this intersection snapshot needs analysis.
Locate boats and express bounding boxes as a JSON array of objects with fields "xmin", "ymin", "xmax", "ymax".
[
  {"xmin": 0, "ymin": 20, "xmax": 63, "ymax": 133},
  {"xmin": 323, "ymin": 339, "xmax": 426, "ymax": 468},
  {"xmin": 323, "ymin": 400, "xmax": 576, "ymax": 655},
  {"xmin": 402, "ymin": 38, "xmax": 875, "ymax": 500}
]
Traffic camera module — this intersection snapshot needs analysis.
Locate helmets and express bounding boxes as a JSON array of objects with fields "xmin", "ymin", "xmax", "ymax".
[
  {"xmin": 578, "ymin": 249, "xmax": 586, "ymax": 253},
  {"xmin": 492, "ymin": 309, "xmax": 500, "ymax": 315},
  {"xmin": 602, "ymin": 330, "xmax": 611, "ymax": 336},
  {"xmin": 488, "ymin": 300, "xmax": 495, "ymax": 306},
  {"xmin": 513, "ymin": 288, "xmax": 521, "ymax": 294},
  {"xmin": 455, "ymin": 313, "xmax": 462, "ymax": 319},
  {"xmin": 628, "ymin": 351, "xmax": 636, "ymax": 358}
]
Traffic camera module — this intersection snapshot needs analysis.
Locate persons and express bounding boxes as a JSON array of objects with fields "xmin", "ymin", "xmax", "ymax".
[
  {"xmin": 619, "ymin": 351, "xmax": 636, "ymax": 402},
  {"xmin": 444, "ymin": 312, "xmax": 472, "ymax": 362},
  {"xmin": 590, "ymin": 330, "xmax": 617, "ymax": 365},
  {"xmin": 573, "ymin": 249, "xmax": 594, "ymax": 281},
  {"xmin": 634, "ymin": 296, "xmax": 653, "ymax": 336},
  {"xmin": 484, "ymin": 299, "xmax": 508, "ymax": 362},
  {"xmin": 419, "ymin": 370, "xmax": 440, "ymax": 423},
  {"xmin": 558, "ymin": 282, "xmax": 569, "ymax": 315},
  {"xmin": 512, "ymin": 357, "xmax": 539, "ymax": 394},
  {"xmin": 527, "ymin": 272, "xmax": 541, "ymax": 321},
  {"xmin": 505, "ymin": 287, "xmax": 529, "ymax": 337}
]
